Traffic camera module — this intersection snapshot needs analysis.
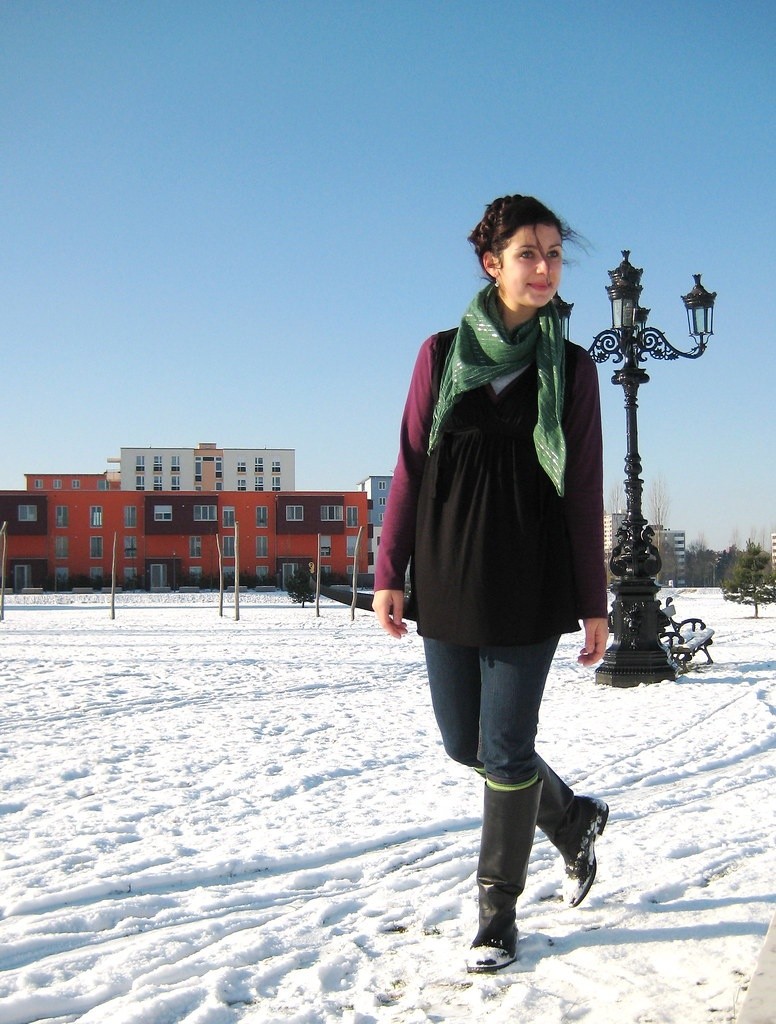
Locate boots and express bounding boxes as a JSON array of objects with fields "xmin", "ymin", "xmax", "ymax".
[
  {"xmin": 475, "ymin": 752, "xmax": 610, "ymax": 905},
  {"xmin": 467, "ymin": 777, "xmax": 541, "ymax": 973}
]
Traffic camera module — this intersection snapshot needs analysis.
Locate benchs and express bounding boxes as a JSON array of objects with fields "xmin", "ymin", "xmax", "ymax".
[{"xmin": 655, "ymin": 596, "xmax": 716, "ymax": 677}]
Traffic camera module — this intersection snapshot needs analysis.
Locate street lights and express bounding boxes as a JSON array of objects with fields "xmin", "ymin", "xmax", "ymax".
[{"xmin": 549, "ymin": 249, "xmax": 717, "ymax": 688}]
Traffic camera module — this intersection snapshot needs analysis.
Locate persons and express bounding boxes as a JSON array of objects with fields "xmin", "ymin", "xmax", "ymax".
[{"xmin": 372, "ymin": 193, "xmax": 609, "ymax": 974}]
